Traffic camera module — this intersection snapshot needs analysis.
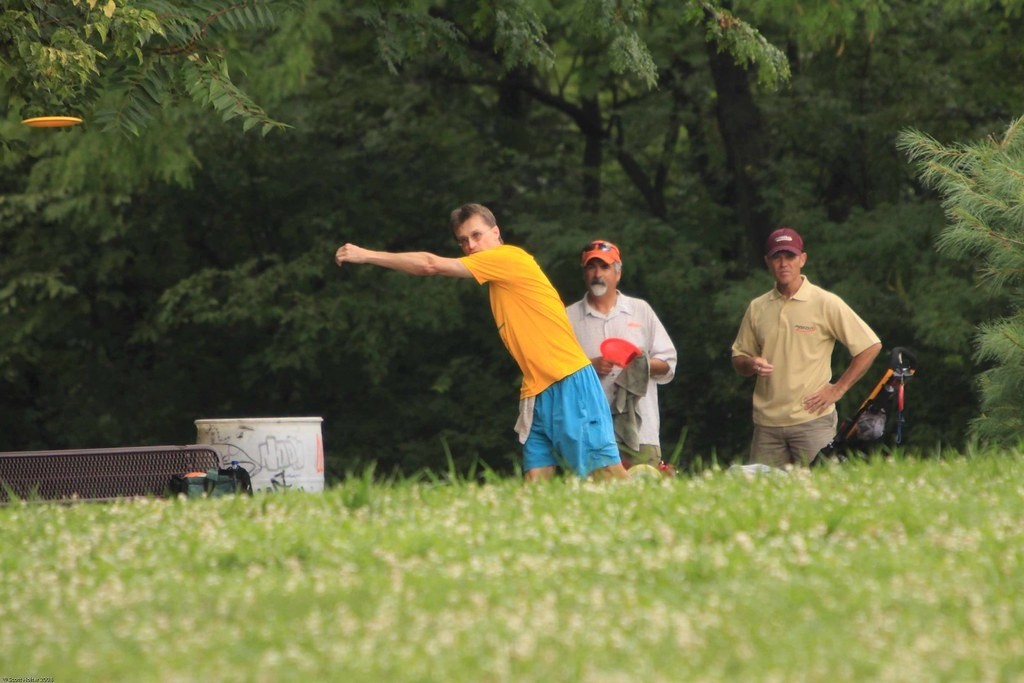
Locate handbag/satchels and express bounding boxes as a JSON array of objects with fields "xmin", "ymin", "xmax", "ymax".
[{"xmin": 170, "ymin": 465, "xmax": 254, "ymax": 498}]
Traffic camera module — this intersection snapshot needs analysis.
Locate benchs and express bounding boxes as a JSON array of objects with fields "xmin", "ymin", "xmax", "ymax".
[{"xmin": 0, "ymin": 442, "xmax": 220, "ymax": 508}]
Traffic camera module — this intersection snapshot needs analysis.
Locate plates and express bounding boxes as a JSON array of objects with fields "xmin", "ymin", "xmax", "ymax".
[
  {"xmin": 601, "ymin": 338, "xmax": 642, "ymax": 366},
  {"xmin": 21, "ymin": 116, "xmax": 83, "ymax": 126}
]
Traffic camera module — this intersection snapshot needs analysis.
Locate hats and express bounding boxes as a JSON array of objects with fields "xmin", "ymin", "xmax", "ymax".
[
  {"xmin": 581, "ymin": 239, "xmax": 622, "ymax": 268},
  {"xmin": 766, "ymin": 228, "xmax": 803, "ymax": 257}
]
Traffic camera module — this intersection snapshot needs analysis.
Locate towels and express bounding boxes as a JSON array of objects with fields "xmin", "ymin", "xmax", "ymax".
[
  {"xmin": 513, "ymin": 394, "xmax": 535, "ymax": 445},
  {"xmin": 610, "ymin": 350, "xmax": 651, "ymax": 452}
]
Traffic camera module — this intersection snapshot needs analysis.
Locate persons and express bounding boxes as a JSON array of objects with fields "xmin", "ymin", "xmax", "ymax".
[
  {"xmin": 730, "ymin": 227, "xmax": 882, "ymax": 472},
  {"xmin": 565, "ymin": 240, "xmax": 677, "ymax": 473},
  {"xmin": 336, "ymin": 203, "xmax": 628, "ymax": 482}
]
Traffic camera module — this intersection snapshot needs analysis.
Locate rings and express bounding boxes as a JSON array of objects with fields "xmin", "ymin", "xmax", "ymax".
[{"xmin": 757, "ymin": 366, "xmax": 761, "ymax": 373}]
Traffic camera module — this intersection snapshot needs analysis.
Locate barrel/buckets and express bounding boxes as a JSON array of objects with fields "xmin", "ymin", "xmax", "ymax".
[{"xmin": 193, "ymin": 417, "xmax": 326, "ymax": 497}]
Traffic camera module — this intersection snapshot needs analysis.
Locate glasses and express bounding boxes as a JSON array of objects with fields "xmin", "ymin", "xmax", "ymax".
[
  {"xmin": 458, "ymin": 226, "xmax": 492, "ymax": 246},
  {"xmin": 584, "ymin": 243, "xmax": 611, "ymax": 251}
]
